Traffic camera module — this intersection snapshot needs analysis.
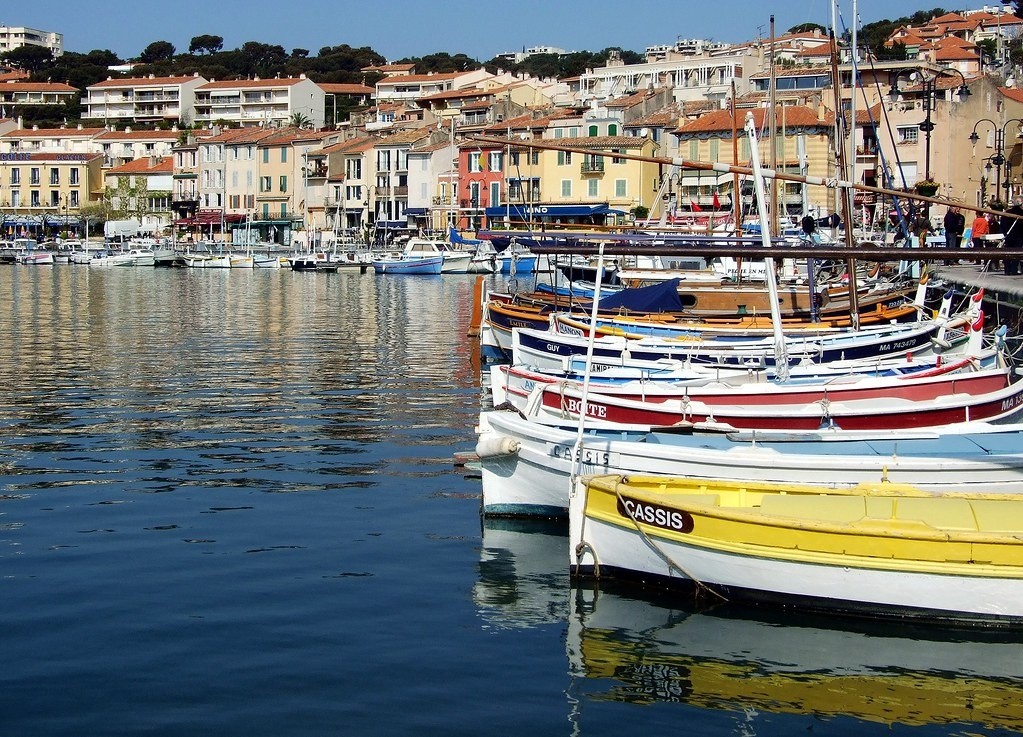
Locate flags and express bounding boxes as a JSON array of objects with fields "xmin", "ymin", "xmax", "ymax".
[
  {"xmin": 478, "ymin": 152, "xmax": 486, "ymax": 170},
  {"xmin": 713, "ymin": 195, "xmax": 720, "ymax": 208},
  {"xmin": 691, "ymin": 201, "xmax": 703, "ymax": 211}
]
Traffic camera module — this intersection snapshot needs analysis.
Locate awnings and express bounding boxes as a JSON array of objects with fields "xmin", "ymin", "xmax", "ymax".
[
  {"xmin": 3, "ymin": 215, "xmax": 82, "ymax": 226},
  {"xmin": 485, "ymin": 204, "xmax": 608, "ymax": 216}
]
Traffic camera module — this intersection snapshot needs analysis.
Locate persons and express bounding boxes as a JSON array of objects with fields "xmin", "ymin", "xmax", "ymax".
[
  {"xmin": 970, "ymin": 197, "xmax": 1023, "ymax": 276},
  {"xmin": 944, "ymin": 205, "xmax": 965, "ymax": 265}
]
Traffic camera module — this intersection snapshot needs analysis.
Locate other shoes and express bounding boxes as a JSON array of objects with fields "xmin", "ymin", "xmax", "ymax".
[
  {"xmin": 973, "ymin": 263, "xmax": 981, "ymax": 265},
  {"xmin": 994, "ymin": 267, "xmax": 1001, "ymax": 271},
  {"xmin": 945, "ymin": 260, "xmax": 962, "ymax": 266},
  {"xmin": 1004, "ymin": 269, "xmax": 1022, "ymax": 275}
]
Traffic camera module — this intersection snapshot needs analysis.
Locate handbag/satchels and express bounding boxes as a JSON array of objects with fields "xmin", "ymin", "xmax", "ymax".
[{"xmin": 968, "ymin": 241, "xmax": 975, "ymax": 248}]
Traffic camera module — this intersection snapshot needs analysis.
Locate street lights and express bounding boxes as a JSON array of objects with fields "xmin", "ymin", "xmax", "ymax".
[
  {"xmin": 59, "ymin": 192, "xmax": 74, "ymax": 231},
  {"xmin": 659, "ymin": 172, "xmax": 683, "ymax": 213},
  {"xmin": 874, "ymin": 165, "xmax": 896, "ymax": 225},
  {"xmin": 885, "ymin": 67, "xmax": 972, "ymax": 221},
  {"xmin": 466, "ymin": 177, "xmax": 488, "ymax": 238},
  {"xmin": 968, "ymin": 118, "xmax": 1022, "ymax": 223},
  {"xmin": 358, "ymin": 184, "xmax": 378, "ymax": 243}
]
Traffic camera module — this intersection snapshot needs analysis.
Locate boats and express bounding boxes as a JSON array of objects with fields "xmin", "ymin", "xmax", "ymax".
[
  {"xmin": 568, "ymin": 474, "xmax": 1023, "ymax": 636},
  {"xmin": 0, "ymin": 0, "xmax": 1023, "ymax": 513}
]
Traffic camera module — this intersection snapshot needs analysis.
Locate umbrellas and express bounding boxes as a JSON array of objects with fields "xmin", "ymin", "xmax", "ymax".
[
  {"xmin": 21, "ymin": 225, "xmax": 26, "ymax": 236},
  {"xmin": 8, "ymin": 225, "xmax": 12, "ymax": 234}
]
